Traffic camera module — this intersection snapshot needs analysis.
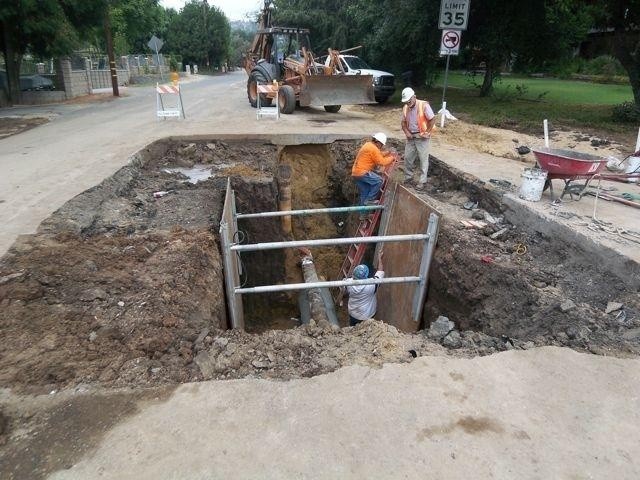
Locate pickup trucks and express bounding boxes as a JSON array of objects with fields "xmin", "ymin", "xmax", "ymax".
[{"xmin": 316, "ymin": 53, "xmax": 396, "ymax": 104}]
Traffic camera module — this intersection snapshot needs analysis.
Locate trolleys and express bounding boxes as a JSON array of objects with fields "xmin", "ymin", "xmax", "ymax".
[{"xmin": 527, "ymin": 147, "xmax": 640, "ymax": 204}]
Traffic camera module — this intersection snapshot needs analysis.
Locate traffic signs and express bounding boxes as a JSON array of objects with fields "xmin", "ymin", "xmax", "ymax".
[{"xmin": 438, "ymin": 0, "xmax": 470, "ymax": 30}]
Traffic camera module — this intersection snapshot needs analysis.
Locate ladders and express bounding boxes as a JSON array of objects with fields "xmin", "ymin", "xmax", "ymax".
[{"xmin": 336, "ymin": 157, "xmax": 396, "ymax": 310}]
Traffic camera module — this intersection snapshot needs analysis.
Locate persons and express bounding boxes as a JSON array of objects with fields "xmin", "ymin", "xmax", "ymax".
[
  {"xmin": 400, "ymin": 87, "xmax": 436, "ymax": 191},
  {"xmin": 341, "ymin": 249, "xmax": 384, "ymax": 326},
  {"xmin": 350, "ymin": 132, "xmax": 395, "ymax": 219}
]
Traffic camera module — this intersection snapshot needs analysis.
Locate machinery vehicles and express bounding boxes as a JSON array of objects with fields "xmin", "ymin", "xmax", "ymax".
[{"xmin": 242, "ymin": 20, "xmax": 379, "ymax": 114}]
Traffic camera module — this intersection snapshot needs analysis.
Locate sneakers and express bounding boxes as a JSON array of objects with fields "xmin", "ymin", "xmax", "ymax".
[
  {"xmin": 359, "ymin": 199, "xmax": 381, "ymax": 221},
  {"xmin": 402, "ymin": 174, "xmax": 426, "ymax": 191}
]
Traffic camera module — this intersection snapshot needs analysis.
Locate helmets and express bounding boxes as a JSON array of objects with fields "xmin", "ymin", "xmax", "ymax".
[
  {"xmin": 373, "ymin": 131, "xmax": 387, "ymax": 146},
  {"xmin": 401, "ymin": 87, "xmax": 415, "ymax": 102},
  {"xmin": 351, "ymin": 264, "xmax": 371, "ymax": 281}
]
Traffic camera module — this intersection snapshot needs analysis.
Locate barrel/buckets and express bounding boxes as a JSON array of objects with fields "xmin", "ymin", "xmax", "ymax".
[{"xmin": 520, "ymin": 165, "xmax": 548, "ymax": 201}]
